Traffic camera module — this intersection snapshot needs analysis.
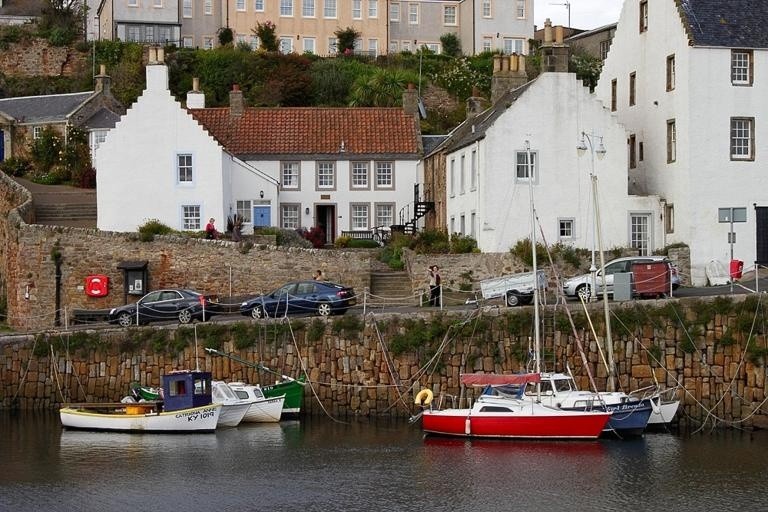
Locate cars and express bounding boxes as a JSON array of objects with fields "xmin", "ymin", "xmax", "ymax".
[
  {"xmin": 108, "ymin": 287, "xmax": 221, "ymax": 328},
  {"xmin": 239, "ymin": 279, "xmax": 358, "ymax": 320}
]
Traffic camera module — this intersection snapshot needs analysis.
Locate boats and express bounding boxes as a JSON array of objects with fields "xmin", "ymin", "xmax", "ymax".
[
  {"xmin": 59, "ymin": 418, "xmax": 301, "ymax": 494},
  {"xmin": 58, "ymin": 369, "xmax": 306, "ymax": 430}
]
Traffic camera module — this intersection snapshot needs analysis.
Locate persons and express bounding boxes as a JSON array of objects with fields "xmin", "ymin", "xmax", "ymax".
[
  {"xmin": 231, "ymin": 221, "xmax": 242, "ymax": 242},
  {"xmin": 427, "ymin": 265, "xmax": 441, "ymax": 307},
  {"xmin": 205, "ymin": 218, "xmax": 217, "ymax": 239},
  {"xmin": 314, "ymin": 270, "xmax": 324, "ymax": 281}
]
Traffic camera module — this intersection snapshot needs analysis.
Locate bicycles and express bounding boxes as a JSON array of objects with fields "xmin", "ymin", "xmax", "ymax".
[{"xmin": 370, "ymin": 225, "xmax": 394, "ymax": 246}]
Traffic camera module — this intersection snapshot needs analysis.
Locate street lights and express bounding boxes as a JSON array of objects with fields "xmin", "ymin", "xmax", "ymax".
[
  {"xmin": 577, "ymin": 130, "xmax": 608, "ymax": 301},
  {"xmin": 54, "ymin": 251, "xmax": 62, "ymax": 325}
]
